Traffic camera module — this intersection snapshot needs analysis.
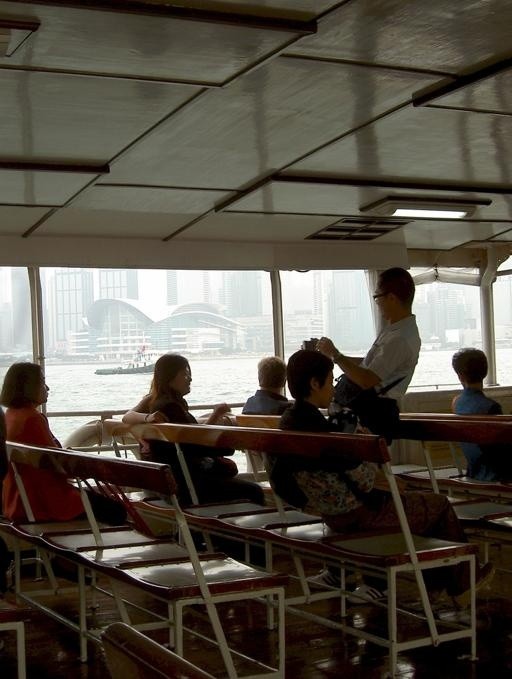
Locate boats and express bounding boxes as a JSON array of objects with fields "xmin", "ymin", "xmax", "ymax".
[{"xmin": 95, "ymin": 363, "xmax": 156, "ymax": 375}]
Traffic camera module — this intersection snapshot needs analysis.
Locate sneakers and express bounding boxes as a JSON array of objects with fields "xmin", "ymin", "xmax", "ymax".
[
  {"xmin": 347, "ymin": 583, "xmax": 400, "ymax": 605},
  {"xmin": 305, "ymin": 567, "xmax": 357, "ymax": 591}
]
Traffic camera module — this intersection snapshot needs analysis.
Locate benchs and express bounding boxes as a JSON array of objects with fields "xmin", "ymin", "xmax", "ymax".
[{"xmin": 0, "ymin": 401, "xmax": 512, "ymax": 678}]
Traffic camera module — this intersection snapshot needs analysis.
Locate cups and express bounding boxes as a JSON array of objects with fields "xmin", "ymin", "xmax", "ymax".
[{"xmin": 301, "ymin": 341, "xmax": 314, "ymax": 351}]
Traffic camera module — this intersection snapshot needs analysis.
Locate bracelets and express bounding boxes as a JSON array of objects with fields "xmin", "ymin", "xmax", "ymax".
[
  {"xmin": 143, "ymin": 413, "xmax": 148, "ymax": 423},
  {"xmin": 333, "ymin": 354, "xmax": 344, "ymax": 364}
]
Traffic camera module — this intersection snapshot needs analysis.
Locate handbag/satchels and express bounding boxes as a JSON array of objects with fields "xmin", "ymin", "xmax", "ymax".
[
  {"xmin": 332, "ymin": 375, "xmax": 400, "ymax": 448},
  {"xmin": 374, "ymin": 469, "xmax": 408, "ymax": 499}
]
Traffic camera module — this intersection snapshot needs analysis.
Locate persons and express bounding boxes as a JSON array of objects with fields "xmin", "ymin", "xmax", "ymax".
[
  {"xmin": 305, "ymin": 266, "xmax": 420, "ymax": 604},
  {"xmin": 450, "ymin": 351, "xmax": 512, "ymax": 483},
  {"xmin": 240, "ymin": 357, "xmax": 293, "ymax": 415},
  {"xmin": 1, "ymin": 362, "xmax": 127, "ymax": 584},
  {"xmin": 145, "ymin": 352, "xmax": 264, "ymax": 557},
  {"xmin": 0, "ymin": 409, "xmax": 15, "ymax": 590},
  {"xmin": 266, "ymin": 348, "xmax": 496, "ymax": 612},
  {"xmin": 123, "ymin": 379, "xmax": 238, "ymax": 478}
]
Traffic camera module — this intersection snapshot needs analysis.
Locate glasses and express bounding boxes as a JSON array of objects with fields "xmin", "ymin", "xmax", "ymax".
[{"xmin": 372, "ymin": 292, "xmax": 383, "ymax": 305}]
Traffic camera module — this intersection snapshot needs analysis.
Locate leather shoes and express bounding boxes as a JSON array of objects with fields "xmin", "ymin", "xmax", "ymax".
[{"xmin": 449, "ymin": 560, "xmax": 496, "ymax": 610}]
